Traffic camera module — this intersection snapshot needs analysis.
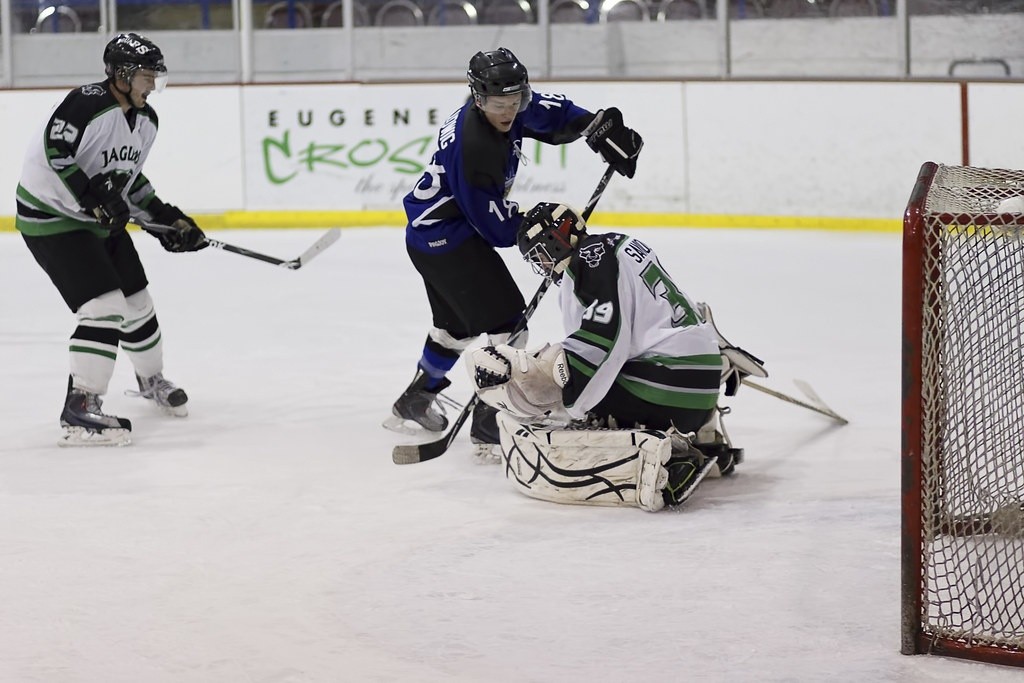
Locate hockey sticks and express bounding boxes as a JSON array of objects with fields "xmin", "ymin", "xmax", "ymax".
[
  {"xmin": 739, "ymin": 378, "xmax": 850, "ymax": 425},
  {"xmin": 126, "ymin": 215, "xmax": 342, "ymax": 271},
  {"xmin": 387, "ymin": 162, "xmax": 615, "ymax": 466}
]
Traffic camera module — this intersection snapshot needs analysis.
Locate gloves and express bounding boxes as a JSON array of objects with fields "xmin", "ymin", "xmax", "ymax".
[
  {"xmin": 147, "ymin": 205, "xmax": 209, "ymax": 253},
  {"xmin": 579, "ymin": 107, "xmax": 644, "ymax": 179},
  {"xmin": 85, "ymin": 169, "xmax": 132, "ymax": 238}
]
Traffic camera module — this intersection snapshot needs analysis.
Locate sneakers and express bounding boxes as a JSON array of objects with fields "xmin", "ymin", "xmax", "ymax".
[
  {"xmin": 470, "ymin": 402, "xmax": 503, "ymax": 462},
  {"xmin": 705, "ymin": 443, "xmax": 736, "ymax": 475},
  {"xmin": 664, "ymin": 450, "xmax": 720, "ymax": 506},
  {"xmin": 59, "ymin": 375, "xmax": 132, "ymax": 446},
  {"xmin": 382, "ymin": 363, "xmax": 463, "ymax": 438},
  {"xmin": 122, "ymin": 371, "xmax": 189, "ymax": 417}
]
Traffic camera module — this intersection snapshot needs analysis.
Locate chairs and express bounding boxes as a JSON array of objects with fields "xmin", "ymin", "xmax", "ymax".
[{"xmin": 21, "ymin": 0, "xmax": 881, "ymax": 33}]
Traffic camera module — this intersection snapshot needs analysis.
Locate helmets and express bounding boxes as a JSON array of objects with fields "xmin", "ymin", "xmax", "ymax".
[
  {"xmin": 104, "ymin": 33, "xmax": 167, "ymax": 73},
  {"xmin": 517, "ymin": 202, "xmax": 586, "ymax": 287},
  {"xmin": 466, "ymin": 46, "xmax": 529, "ymax": 96}
]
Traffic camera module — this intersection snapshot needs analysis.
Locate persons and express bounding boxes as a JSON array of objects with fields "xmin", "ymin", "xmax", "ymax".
[
  {"xmin": 460, "ymin": 203, "xmax": 769, "ymax": 512},
  {"xmin": 382, "ymin": 47, "xmax": 644, "ymax": 469},
  {"xmin": 14, "ymin": 33, "xmax": 209, "ymax": 447}
]
